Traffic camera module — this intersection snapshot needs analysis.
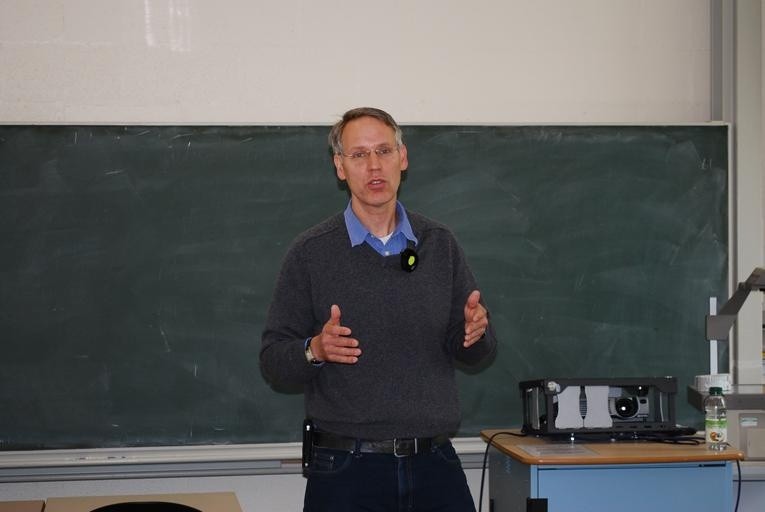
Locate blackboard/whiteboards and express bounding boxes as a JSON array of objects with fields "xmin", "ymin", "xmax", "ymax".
[{"xmin": 0, "ymin": 120, "xmax": 735, "ymax": 468}]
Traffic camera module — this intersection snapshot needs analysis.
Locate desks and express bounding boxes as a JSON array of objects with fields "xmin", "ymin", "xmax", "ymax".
[{"xmin": 482, "ymin": 427, "xmax": 746, "ymax": 512}]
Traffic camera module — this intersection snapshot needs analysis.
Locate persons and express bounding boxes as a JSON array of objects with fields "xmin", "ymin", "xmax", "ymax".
[{"xmin": 260, "ymin": 109, "xmax": 498, "ymax": 511}]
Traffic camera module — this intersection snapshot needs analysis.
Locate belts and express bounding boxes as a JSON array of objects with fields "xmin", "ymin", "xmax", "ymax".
[{"xmin": 313, "ymin": 436, "xmax": 447, "ymax": 459}]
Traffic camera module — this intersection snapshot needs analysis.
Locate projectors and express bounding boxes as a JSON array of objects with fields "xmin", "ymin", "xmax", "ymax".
[{"xmin": 519, "ymin": 375, "xmax": 677, "ymax": 445}]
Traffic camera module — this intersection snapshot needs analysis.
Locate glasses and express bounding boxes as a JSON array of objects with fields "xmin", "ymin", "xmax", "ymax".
[{"xmin": 347, "ymin": 147, "xmax": 396, "ymax": 167}]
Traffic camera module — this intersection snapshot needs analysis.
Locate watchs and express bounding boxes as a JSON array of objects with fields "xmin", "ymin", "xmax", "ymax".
[{"xmin": 305, "ymin": 338, "xmax": 323, "ymax": 365}]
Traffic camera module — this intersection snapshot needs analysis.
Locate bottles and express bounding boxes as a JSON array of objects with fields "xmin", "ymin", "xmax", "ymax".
[{"xmin": 703, "ymin": 385, "xmax": 729, "ymax": 452}]
